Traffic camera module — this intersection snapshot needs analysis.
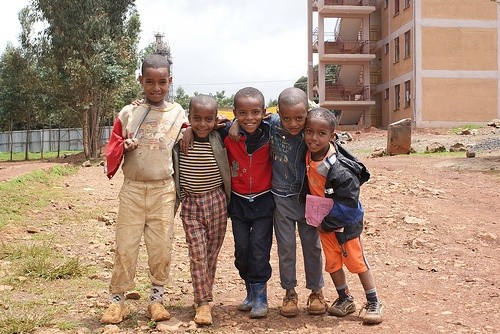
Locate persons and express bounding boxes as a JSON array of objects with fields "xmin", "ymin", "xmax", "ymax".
[{"xmin": 99, "ymin": 55, "xmax": 382, "ymax": 323}]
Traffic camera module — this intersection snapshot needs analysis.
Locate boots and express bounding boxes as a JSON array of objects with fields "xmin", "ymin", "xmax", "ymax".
[
  {"xmin": 250, "ymin": 282, "xmax": 268, "ymax": 316},
  {"xmin": 238, "ymin": 282, "xmax": 255, "ymax": 310}
]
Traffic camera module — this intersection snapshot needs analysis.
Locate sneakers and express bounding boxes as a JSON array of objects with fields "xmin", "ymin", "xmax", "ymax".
[
  {"xmin": 363, "ymin": 301, "xmax": 384, "ymax": 322},
  {"xmin": 194, "ymin": 305, "xmax": 212, "ymax": 324},
  {"xmin": 280, "ymin": 294, "xmax": 299, "ymax": 315},
  {"xmin": 307, "ymin": 292, "xmax": 328, "ymax": 313},
  {"xmin": 102, "ymin": 302, "xmax": 130, "ymax": 323},
  {"xmin": 148, "ymin": 303, "xmax": 170, "ymax": 322},
  {"xmin": 328, "ymin": 296, "xmax": 356, "ymax": 316}
]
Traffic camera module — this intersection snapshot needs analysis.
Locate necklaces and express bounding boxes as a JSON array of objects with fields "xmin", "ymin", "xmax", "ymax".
[{"xmin": 306, "ymin": 146, "xmax": 328, "ymax": 170}]
